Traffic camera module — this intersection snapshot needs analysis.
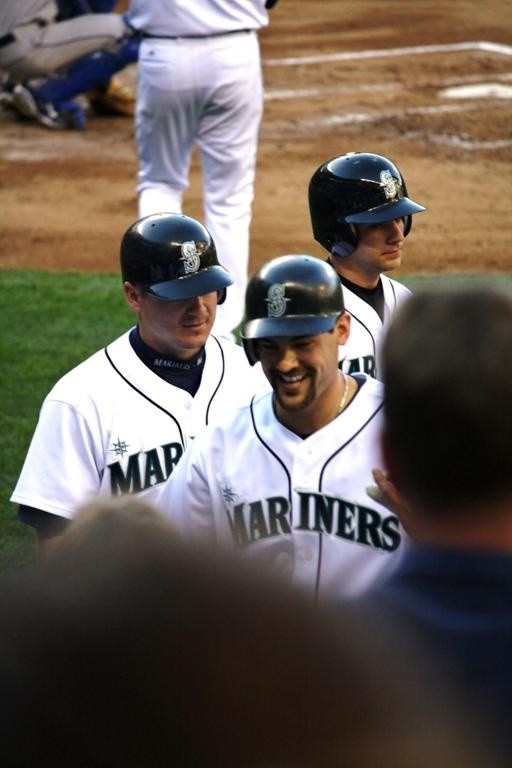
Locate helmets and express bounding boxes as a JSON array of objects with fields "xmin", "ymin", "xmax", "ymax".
[
  {"xmin": 119, "ymin": 210, "xmax": 236, "ymax": 303},
  {"xmin": 307, "ymin": 149, "xmax": 428, "ymax": 240},
  {"xmin": 241, "ymin": 250, "xmax": 347, "ymax": 344}
]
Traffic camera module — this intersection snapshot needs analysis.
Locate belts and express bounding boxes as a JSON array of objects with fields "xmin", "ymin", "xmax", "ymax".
[{"xmin": 141, "ymin": 28, "xmax": 253, "ymax": 39}]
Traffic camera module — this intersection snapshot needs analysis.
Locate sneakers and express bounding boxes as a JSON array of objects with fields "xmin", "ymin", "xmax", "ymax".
[{"xmin": 12, "ymin": 82, "xmax": 74, "ymax": 131}]
